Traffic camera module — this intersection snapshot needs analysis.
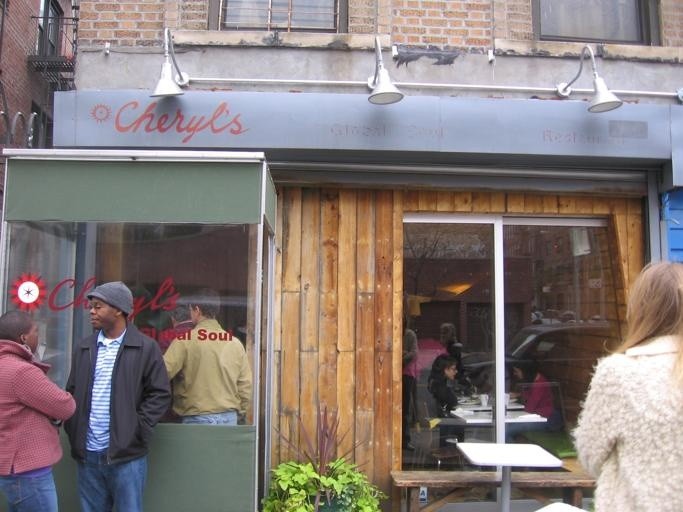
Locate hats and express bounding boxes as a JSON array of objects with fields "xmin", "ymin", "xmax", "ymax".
[{"xmin": 87, "ymin": 281, "xmax": 132, "ymax": 314}]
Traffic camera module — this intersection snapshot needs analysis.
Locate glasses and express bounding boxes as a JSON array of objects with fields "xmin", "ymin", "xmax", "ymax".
[{"xmin": 447, "ymin": 367, "xmax": 458, "ymax": 372}]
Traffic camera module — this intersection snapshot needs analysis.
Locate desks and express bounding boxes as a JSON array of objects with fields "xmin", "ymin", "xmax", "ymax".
[
  {"xmin": 450, "ymin": 392, "xmax": 547, "ymax": 442},
  {"xmin": 455, "ymin": 442, "xmax": 563, "ymax": 511}
]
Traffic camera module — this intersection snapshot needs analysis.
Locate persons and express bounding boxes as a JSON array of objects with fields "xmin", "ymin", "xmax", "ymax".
[
  {"xmin": 162, "ymin": 285, "xmax": 254, "ymax": 428},
  {"xmin": 568, "ymin": 257, "xmax": 682, "ymax": 512},
  {"xmin": 57, "ymin": 279, "xmax": 173, "ymax": 511},
  {"xmin": 156, "ymin": 305, "xmax": 195, "ymax": 357},
  {"xmin": 0, "ymin": 310, "xmax": 77, "ymax": 511},
  {"xmin": 438, "ymin": 320, "xmax": 466, "ymax": 356},
  {"xmin": 500, "ymin": 351, "xmax": 558, "ymax": 444},
  {"xmin": 424, "ymin": 352, "xmax": 460, "ymax": 420},
  {"xmin": 401, "ymin": 311, "xmax": 419, "ymax": 452}
]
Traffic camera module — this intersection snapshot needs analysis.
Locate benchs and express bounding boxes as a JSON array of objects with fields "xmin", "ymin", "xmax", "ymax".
[{"xmin": 390, "ymin": 470, "xmax": 597, "ymax": 512}]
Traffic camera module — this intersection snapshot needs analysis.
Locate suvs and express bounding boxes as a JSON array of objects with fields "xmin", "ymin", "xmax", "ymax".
[{"xmin": 458, "ymin": 319, "xmax": 619, "ymax": 423}]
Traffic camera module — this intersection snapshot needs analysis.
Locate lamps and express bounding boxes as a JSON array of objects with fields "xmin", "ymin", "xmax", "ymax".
[
  {"xmin": 366, "ymin": 35, "xmax": 403, "ymax": 105},
  {"xmin": 557, "ymin": 43, "xmax": 622, "ymax": 114},
  {"xmin": 149, "ymin": 28, "xmax": 189, "ymax": 99}
]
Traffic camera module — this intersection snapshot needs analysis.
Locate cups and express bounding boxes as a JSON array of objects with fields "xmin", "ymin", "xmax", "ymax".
[{"xmin": 480, "ymin": 394, "xmax": 488, "ymax": 408}]
